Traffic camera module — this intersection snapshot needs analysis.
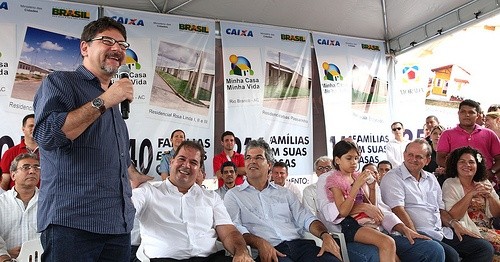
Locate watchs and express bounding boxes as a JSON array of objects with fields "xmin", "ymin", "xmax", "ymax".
[
  {"xmin": 488, "ymin": 168, "xmax": 496, "ymax": 175},
  {"xmin": 90, "ymin": 97, "xmax": 107, "ymax": 116}
]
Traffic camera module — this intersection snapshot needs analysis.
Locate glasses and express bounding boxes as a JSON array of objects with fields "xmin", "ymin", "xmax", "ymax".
[
  {"xmin": 317, "ymin": 166, "xmax": 331, "ymax": 171},
  {"xmin": 15, "ymin": 165, "xmax": 41, "ymax": 172},
  {"xmin": 86, "ymin": 36, "xmax": 130, "ymax": 50},
  {"xmin": 392, "ymin": 128, "xmax": 402, "ymax": 131}
]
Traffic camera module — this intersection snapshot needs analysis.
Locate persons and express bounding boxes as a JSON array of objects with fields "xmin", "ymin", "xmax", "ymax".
[
  {"xmin": 440, "ymin": 146, "xmax": 500, "ymax": 262},
  {"xmin": 362, "ymin": 99, "xmax": 500, "ymax": 230},
  {"xmin": 158, "ymin": 129, "xmax": 303, "ymax": 204},
  {"xmin": 32, "ymin": 17, "xmax": 155, "ymax": 262},
  {"xmin": 325, "ymin": 139, "xmax": 400, "ymax": 262},
  {"xmin": 223, "ymin": 140, "xmax": 342, "ymax": 262},
  {"xmin": 379, "ymin": 138, "xmax": 495, "ymax": 262},
  {"xmin": 315, "ymin": 138, "xmax": 446, "ymax": 262},
  {"xmin": 0, "ymin": 115, "xmax": 49, "ymax": 262},
  {"xmin": 129, "ymin": 141, "xmax": 257, "ymax": 262}
]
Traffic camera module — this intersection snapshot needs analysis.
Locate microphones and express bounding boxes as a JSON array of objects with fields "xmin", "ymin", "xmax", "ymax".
[{"xmin": 118, "ymin": 64, "xmax": 130, "ymax": 119}]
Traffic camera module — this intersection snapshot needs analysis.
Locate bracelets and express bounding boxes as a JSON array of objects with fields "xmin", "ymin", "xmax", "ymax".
[
  {"xmin": 320, "ymin": 231, "xmax": 329, "ymax": 239},
  {"xmin": 449, "ymin": 219, "xmax": 459, "ymax": 227}
]
